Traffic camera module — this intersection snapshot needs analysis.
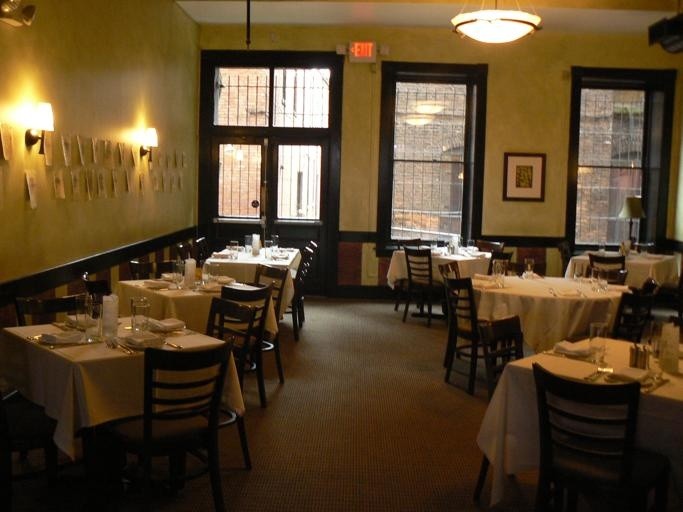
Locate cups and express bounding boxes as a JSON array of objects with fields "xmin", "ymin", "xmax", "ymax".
[
  {"xmin": 230, "ymin": 233, "xmax": 280, "ymax": 260},
  {"xmin": 590, "ymin": 268, "xmax": 600, "ymax": 290},
  {"xmin": 640, "ymin": 245, "xmax": 648, "ymax": 256},
  {"xmin": 492, "ymin": 264, "xmax": 506, "ymax": 288},
  {"xmin": 429, "ymin": 234, "xmax": 476, "ymax": 258},
  {"xmin": 168, "ymin": 257, "xmax": 225, "ymax": 291},
  {"xmin": 598, "ymin": 238, "xmax": 606, "ymax": 253},
  {"xmin": 522, "ymin": 257, "xmax": 535, "ymax": 281},
  {"xmin": 596, "ymin": 269, "xmax": 609, "ymax": 296},
  {"xmin": 74, "ymin": 293, "xmax": 150, "ymax": 344},
  {"xmin": 588, "ymin": 320, "xmax": 606, "ymax": 364}
]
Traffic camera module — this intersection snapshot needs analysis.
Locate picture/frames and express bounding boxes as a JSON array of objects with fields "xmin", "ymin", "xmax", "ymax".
[{"xmin": 502, "ymin": 150, "xmax": 548, "ymax": 201}]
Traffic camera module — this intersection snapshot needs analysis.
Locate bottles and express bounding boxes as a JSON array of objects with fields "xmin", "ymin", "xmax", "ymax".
[{"xmin": 628, "ymin": 343, "xmax": 647, "ymax": 369}]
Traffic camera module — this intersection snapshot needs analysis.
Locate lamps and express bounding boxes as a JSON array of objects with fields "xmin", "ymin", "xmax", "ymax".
[
  {"xmin": 136, "ymin": 125, "xmax": 159, "ymax": 156},
  {"xmin": 620, "ymin": 196, "xmax": 648, "ymax": 245},
  {"xmin": 25, "ymin": 103, "xmax": 55, "ymax": 146},
  {"xmin": 450, "ymin": 0, "xmax": 551, "ymax": 44}
]
